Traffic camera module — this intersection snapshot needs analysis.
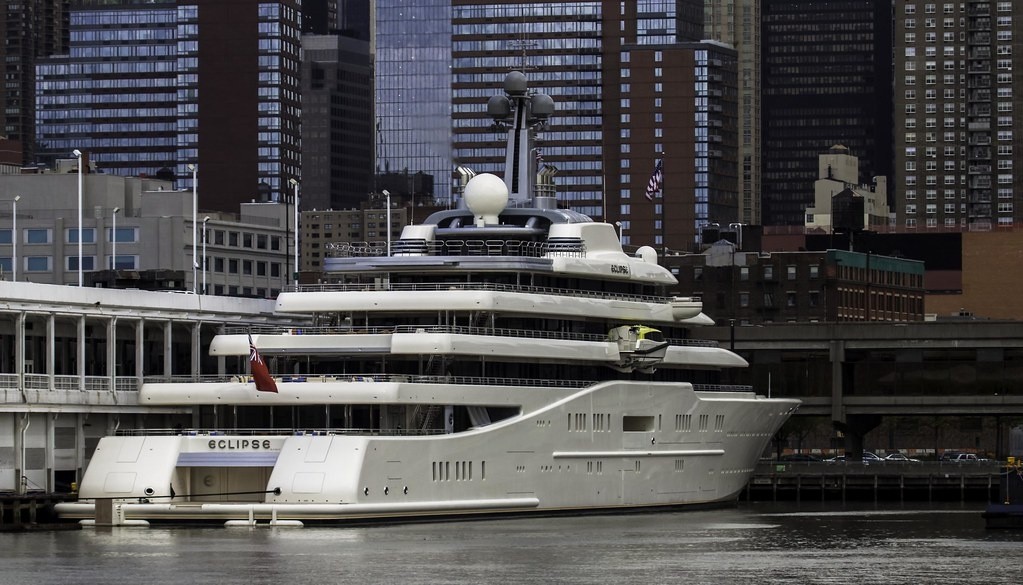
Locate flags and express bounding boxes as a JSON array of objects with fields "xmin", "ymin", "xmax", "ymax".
[
  {"xmin": 248, "ymin": 336, "xmax": 279, "ymax": 393},
  {"xmin": 644, "ymin": 160, "xmax": 663, "ymax": 202}
]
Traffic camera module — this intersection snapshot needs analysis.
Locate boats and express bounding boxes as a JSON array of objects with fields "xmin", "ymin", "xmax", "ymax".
[
  {"xmin": 606, "ymin": 324, "xmax": 670, "ymax": 366},
  {"xmin": 36, "ymin": 11, "xmax": 804, "ymax": 527}
]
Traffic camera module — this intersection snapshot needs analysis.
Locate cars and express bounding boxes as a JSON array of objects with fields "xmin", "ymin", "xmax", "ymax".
[
  {"xmin": 823, "ymin": 455, "xmax": 846, "ymax": 462},
  {"xmin": 863, "ymin": 451, "xmax": 883, "ymax": 460},
  {"xmin": 945, "ymin": 453, "xmax": 986, "ymax": 460},
  {"xmin": 884, "ymin": 452, "xmax": 918, "ymax": 461}
]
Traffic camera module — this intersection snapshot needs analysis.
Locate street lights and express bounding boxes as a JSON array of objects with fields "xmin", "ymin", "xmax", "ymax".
[
  {"xmin": 188, "ymin": 164, "xmax": 197, "ymax": 293},
  {"xmin": 73, "ymin": 149, "xmax": 83, "ymax": 285},
  {"xmin": 289, "ymin": 178, "xmax": 299, "ymax": 292},
  {"xmin": 203, "ymin": 216, "xmax": 210, "ymax": 294},
  {"xmin": 12, "ymin": 196, "xmax": 21, "ymax": 280},
  {"xmin": 615, "ymin": 221, "xmax": 622, "ymax": 247},
  {"xmin": 113, "ymin": 207, "xmax": 120, "ymax": 270},
  {"xmin": 383, "ymin": 190, "xmax": 391, "ymax": 257}
]
